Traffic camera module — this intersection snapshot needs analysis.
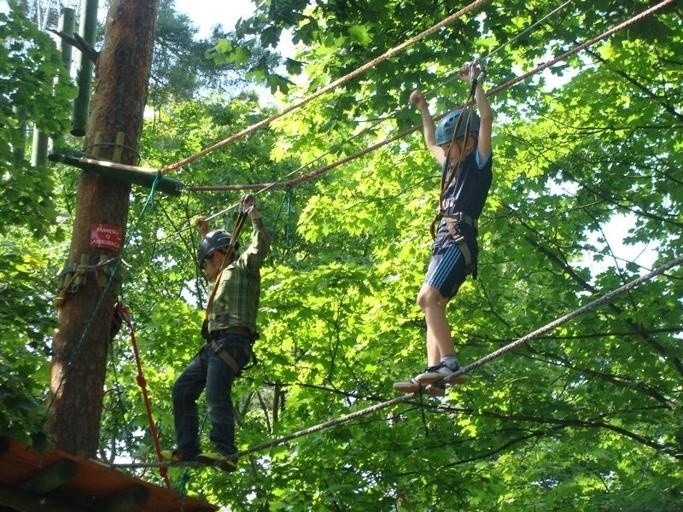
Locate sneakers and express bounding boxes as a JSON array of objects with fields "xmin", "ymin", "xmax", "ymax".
[
  {"xmin": 196, "ymin": 452, "xmax": 238, "ymax": 473},
  {"xmin": 414, "ymin": 360, "xmax": 466, "ymax": 386},
  {"xmin": 391, "ymin": 379, "xmax": 447, "ymax": 398},
  {"xmin": 159, "ymin": 448, "xmax": 201, "ymax": 467}
]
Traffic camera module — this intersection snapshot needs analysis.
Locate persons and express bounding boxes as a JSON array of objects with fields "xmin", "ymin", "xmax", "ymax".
[
  {"xmin": 160, "ymin": 193, "xmax": 271, "ymax": 472},
  {"xmin": 392, "ymin": 61, "xmax": 493, "ymax": 397}
]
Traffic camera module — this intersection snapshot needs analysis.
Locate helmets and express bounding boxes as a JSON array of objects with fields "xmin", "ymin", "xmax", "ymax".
[
  {"xmin": 196, "ymin": 228, "xmax": 240, "ymax": 268},
  {"xmin": 434, "ymin": 108, "xmax": 482, "ymax": 147}
]
